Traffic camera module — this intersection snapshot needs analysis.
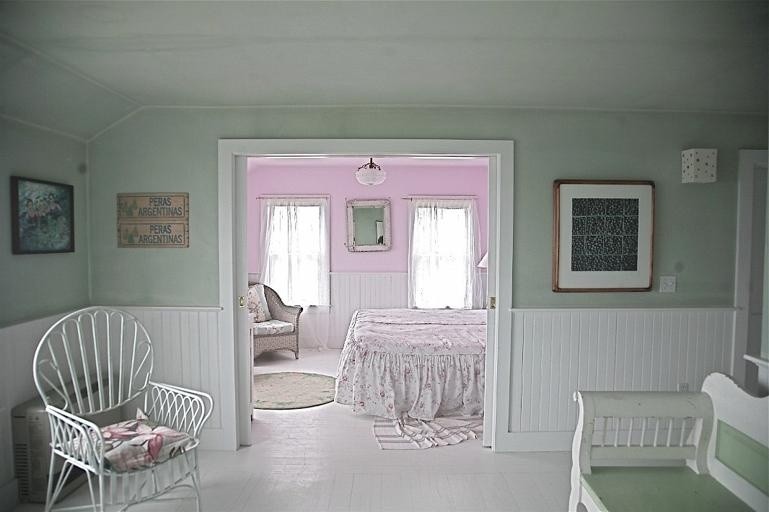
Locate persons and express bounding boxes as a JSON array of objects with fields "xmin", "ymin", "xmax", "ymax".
[{"xmin": 24, "ymin": 193, "xmax": 63, "ymax": 224}]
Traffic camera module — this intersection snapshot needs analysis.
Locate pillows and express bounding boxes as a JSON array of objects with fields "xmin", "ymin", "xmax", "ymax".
[{"xmin": 248, "ymin": 285, "xmax": 273, "ymax": 322}]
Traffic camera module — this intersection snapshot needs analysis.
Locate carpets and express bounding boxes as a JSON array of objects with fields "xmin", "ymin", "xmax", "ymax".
[{"xmin": 253, "ymin": 371, "xmax": 336, "ymax": 409}]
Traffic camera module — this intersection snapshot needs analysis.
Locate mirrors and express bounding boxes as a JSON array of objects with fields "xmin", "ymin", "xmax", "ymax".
[{"xmin": 346, "ymin": 199, "xmax": 392, "ymax": 252}]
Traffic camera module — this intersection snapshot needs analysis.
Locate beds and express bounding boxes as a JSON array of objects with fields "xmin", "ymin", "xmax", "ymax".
[{"xmin": 334, "ymin": 308, "xmax": 487, "ymax": 420}]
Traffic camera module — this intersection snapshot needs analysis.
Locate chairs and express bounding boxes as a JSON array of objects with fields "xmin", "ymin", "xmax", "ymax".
[
  {"xmin": 32, "ymin": 305, "xmax": 213, "ymax": 512},
  {"xmin": 249, "ymin": 282, "xmax": 303, "ymax": 360}
]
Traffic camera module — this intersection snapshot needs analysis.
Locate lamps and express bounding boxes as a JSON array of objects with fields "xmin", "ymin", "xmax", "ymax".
[
  {"xmin": 356, "ymin": 158, "xmax": 387, "ymax": 185},
  {"xmin": 681, "ymin": 148, "xmax": 718, "ymax": 184},
  {"xmin": 477, "ymin": 251, "xmax": 488, "ymax": 268}
]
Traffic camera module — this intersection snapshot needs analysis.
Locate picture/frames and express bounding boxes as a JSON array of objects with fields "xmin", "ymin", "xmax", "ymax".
[
  {"xmin": 552, "ymin": 179, "xmax": 655, "ymax": 292},
  {"xmin": 10, "ymin": 175, "xmax": 75, "ymax": 255}
]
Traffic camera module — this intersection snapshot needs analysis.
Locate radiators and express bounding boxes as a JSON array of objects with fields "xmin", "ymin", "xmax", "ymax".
[{"xmin": 11, "ymin": 375, "xmax": 121, "ymax": 504}]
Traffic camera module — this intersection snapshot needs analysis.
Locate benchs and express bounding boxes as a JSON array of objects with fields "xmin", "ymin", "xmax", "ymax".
[{"xmin": 567, "ymin": 372, "xmax": 769, "ymax": 512}]
